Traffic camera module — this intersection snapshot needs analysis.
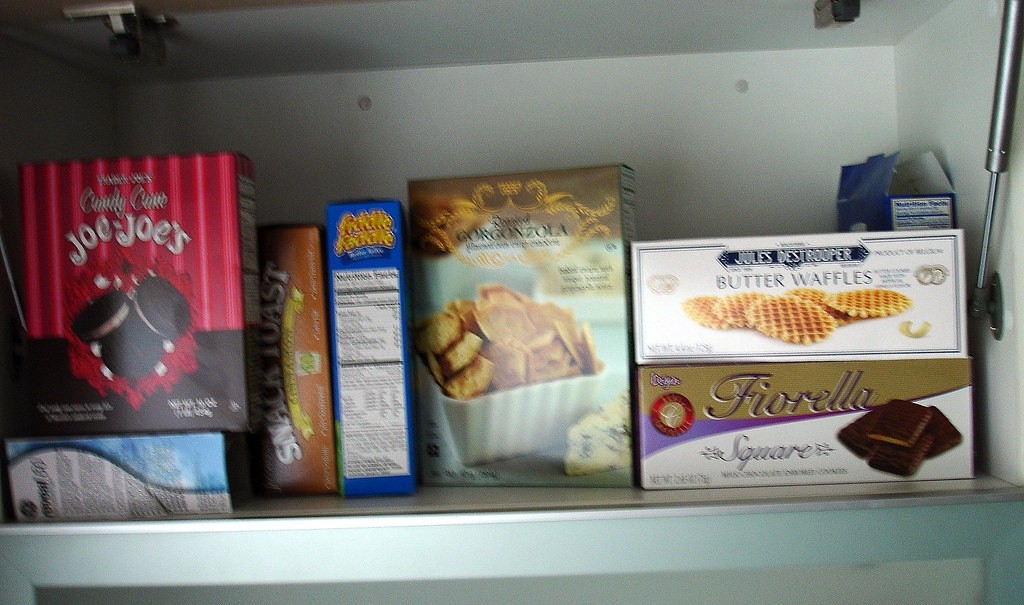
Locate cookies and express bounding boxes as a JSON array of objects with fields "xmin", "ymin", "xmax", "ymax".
[
  {"xmin": 686, "ymin": 288, "xmax": 909, "ymax": 345},
  {"xmin": 417, "ymin": 285, "xmax": 596, "ymax": 397},
  {"xmin": 838, "ymin": 399, "xmax": 961, "ymax": 475},
  {"xmin": 74, "ymin": 277, "xmax": 191, "ymax": 378}
]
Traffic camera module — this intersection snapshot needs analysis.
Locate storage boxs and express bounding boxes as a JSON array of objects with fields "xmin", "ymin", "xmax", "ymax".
[
  {"xmin": 639, "ymin": 360, "xmax": 976, "ymax": 491},
  {"xmin": 837, "ymin": 150, "xmax": 957, "ymax": 230},
  {"xmin": 326, "ymin": 196, "xmax": 416, "ymax": 498},
  {"xmin": 629, "ymin": 229, "xmax": 970, "ymax": 365},
  {"xmin": 6, "ymin": 430, "xmax": 254, "ymax": 522},
  {"xmin": 250, "ymin": 223, "xmax": 339, "ymax": 498},
  {"xmin": 408, "ymin": 163, "xmax": 639, "ymax": 488},
  {"xmin": 17, "ymin": 151, "xmax": 267, "ymax": 437}
]
